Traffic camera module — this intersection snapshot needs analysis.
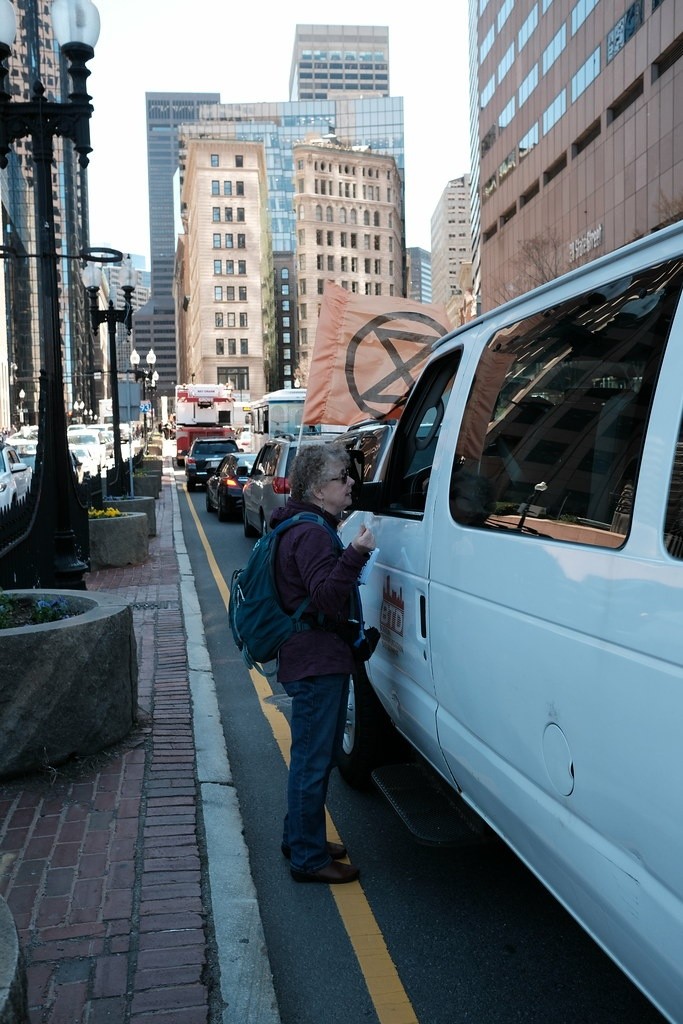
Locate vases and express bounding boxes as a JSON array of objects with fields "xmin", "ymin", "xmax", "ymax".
[
  {"xmin": 0, "ymin": 588, "xmax": 141, "ymax": 780},
  {"xmin": 133, "ymin": 474, "xmax": 160, "ymax": 499},
  {"xmin": 90, "ymin": 511, "xmax": 148, "ymax": 569},
  {"xmin": 104, "ymin": 496, "xmax": 156, "ymax": 536},
  {"xmin": 141, "ymin": 433, "xmax": 164, "ymax": 475}
]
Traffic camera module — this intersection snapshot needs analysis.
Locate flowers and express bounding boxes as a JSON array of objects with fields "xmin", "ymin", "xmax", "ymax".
[
  {"xmin": 0, "ymin": 589, "xmax": 77, "ymax": 628},
  {"xmin": 107, "ymin": 490, "xmax": 139, "ymax": 502},
  {"xmin": 88, "ymin": 505, "xmax": 130, "ymax": 521},
  {"xmin": 133, "ymin": 472, "xmax": 147, "ymax": 479}
]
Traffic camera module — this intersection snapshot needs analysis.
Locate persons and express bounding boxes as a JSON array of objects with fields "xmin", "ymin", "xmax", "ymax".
[
  {"xmin": 268, "ymin": 447, "xmax": 374, "ymax": 883},
  {"xmin": 9, "ymin": 424, "xmax": 17, "ymax": 437}
]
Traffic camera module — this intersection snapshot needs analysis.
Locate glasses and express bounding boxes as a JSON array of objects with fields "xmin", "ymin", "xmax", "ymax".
[{"xmin": 328, "ymin": 469, "xmax": 349, "ymax": 484}]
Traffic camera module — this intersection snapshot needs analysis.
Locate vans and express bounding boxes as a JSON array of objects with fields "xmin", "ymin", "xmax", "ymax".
[{"xmin": 337, "ymin": 220, "xmax": 683, "ymax": 1023}]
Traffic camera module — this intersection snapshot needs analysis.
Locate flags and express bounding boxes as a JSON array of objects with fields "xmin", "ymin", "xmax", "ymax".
[
  {"xmin": 302, "ymin": 282, "xmax": 456, "ymax": 425},
  {"xmin": 450, "ymin": 344, "xmax": 517, "ymax": 461}
]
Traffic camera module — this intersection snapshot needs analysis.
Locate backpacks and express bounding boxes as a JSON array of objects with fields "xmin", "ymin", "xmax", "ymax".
[{"xmin": 228, "ymin": 511, "xmax": 341, "ymax": 669}]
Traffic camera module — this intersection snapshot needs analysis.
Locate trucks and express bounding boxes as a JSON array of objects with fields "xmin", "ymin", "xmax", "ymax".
[{"xmin": 176, "ymin": 382, "xmax": 321, "ymax": 467}]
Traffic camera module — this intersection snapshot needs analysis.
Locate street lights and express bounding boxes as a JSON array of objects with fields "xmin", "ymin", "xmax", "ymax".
[
  {"xmin": 518, "ymin": 482, "xmax": 548, "ymax": 529},
  {"xmin": 144, "ymin": 371, "xmax": 159, "ymax": 432},
  {"xmin": 130, "ymin": 349, "xmax": 156, "ymax": 455},
  {"xmin": 0, "ymin": 2, "xmax": 102, "ymax": 590},
  {"xmin": 80, "ymin": 253, "xmax": 137, "ymax": 498}
]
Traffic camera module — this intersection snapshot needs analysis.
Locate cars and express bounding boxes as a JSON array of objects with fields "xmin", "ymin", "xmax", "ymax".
[
  {"xmin": 206, "ymin": 452, "xmax": 259, "ymax": 522},
  {"xmin": 0, "ymin": 441, "xmax": 33, "ymax": 519},
  {"xmin": 235, "ymin": 431, "xmax": 253, "ymax": 452},
  {"xmin": 4, "ymin": 420, "xmax": 146, "ymax": 486}
]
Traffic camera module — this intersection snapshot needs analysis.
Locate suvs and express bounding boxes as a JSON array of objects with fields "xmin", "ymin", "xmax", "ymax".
[
  {"xmin": 236, "ymin": 430, "xmax": 345, "ymax": 539},
  {"xmin": 331, "ymin": 417, "xmax": 443, "ymax": 482},
  {"xmin": 181, "ymin": 437, "xmax": 244, "ymax": 492}
]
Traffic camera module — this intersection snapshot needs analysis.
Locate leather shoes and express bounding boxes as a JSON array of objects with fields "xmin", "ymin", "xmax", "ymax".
[
  {"xmin": 289, "ymin": 857, "xmax": 357, "ymax": 885},
  {"xmin": 280, "ymin": 837, "xmax": 347, "ymax": 862}
]
